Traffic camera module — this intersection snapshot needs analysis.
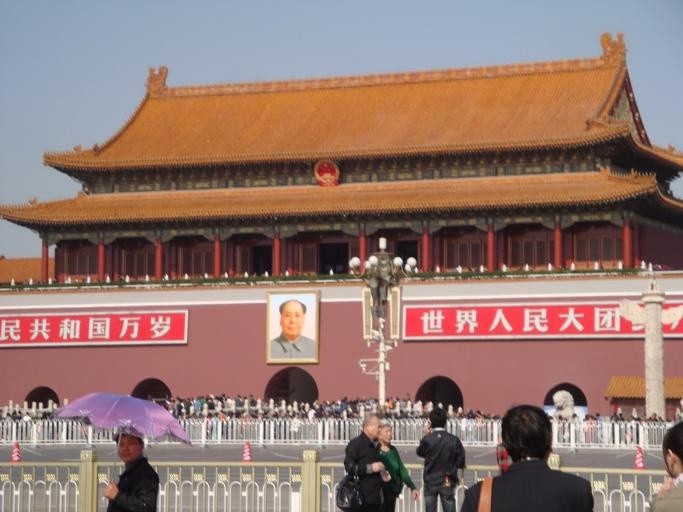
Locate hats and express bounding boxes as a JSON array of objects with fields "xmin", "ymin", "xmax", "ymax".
[{"xmin": 114, "ymin": 426, "xmax": 143, "ymax": 440}]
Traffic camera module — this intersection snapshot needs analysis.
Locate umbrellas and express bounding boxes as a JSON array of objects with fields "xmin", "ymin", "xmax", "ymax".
[{"xmin": 49, "ymin": 390, "xmax": 193, "ymax": 501}]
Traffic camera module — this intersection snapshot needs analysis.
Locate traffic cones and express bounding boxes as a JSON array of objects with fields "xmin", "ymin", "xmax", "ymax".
[
  {"xmin": 241, "ymin": 441, "xmax": 252, "ymax": 464},
  {"xmin": 11, "ymin": 442, "xmax": 21, "ymax": 462},
  {"xmin": 633, "ymin": 446, "xmax": 646, "ymax": 472}
]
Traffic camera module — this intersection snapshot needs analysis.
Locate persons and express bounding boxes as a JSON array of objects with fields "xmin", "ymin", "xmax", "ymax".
[
  {"xmin": 375, "ymin": 424, "xmax": 419, "ymax": 512},
  {"xmin": 648, "ymin": 421, "xmax": 683, "ymax": 512},
  {"xmin": 343, "ymin": 415, "xmax": 391, "ymax": 512},
  {"xmin": 270, "ymin": 300, "xmax": 315, "ymax": 358},
  {"xmin": 161, "ymin": 393, "xmax": 499, "ymax": 440},
  {"xmin": 461, "ymin": 405, "xmax": 594, "ymax": 512},
  {"xmin": 103, "ymin": 426, "xmax": 159, "ymax": 512},
  {"xmin": 416, "ymin": 407, "xmax": 465, "ymax": 512},
  {"xmin": 558, "ymin": 409, "xmax": 663, "ymax": 443},
  {"xmin": 2, "ymin": 411, "xmax": 59, "ymax": 440}
]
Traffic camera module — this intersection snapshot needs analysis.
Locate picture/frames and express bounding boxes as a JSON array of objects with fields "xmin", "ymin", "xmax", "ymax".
[{"xmin": 266, "ymin": 290, "xmax": 322, "ymax": 367}]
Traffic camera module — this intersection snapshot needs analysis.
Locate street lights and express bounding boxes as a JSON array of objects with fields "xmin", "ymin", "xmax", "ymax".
[{"xmin": 346, "ymin": 236, "xmax": 417, "ymax": 405}]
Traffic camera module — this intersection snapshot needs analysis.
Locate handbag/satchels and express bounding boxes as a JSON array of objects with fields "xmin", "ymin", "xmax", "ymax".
[{"xmin": 335, "ymin": 475, "xmax": 362, "ymax": 512}]
